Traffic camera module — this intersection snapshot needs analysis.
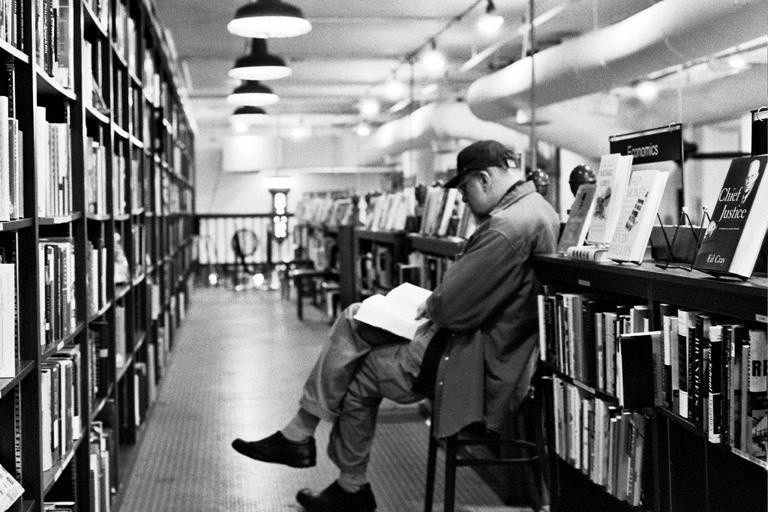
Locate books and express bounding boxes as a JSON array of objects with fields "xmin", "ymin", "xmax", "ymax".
[
  {"xmin": 88, "ymin": 351, "xmax": 147, "ymax": 511},
  {"xmin": 607, "ymin": 170, "xmax": 670, "ymax": 264},
  {"xmin": 2, "ymin": 0, "xmax": 74, "ymax": 92},
  {"xmin": 660, "ymin": 304, "xmax": 766, "ymax": 458},
  {"xmin": 89, "ymin": 217, "xmax": 194, "ymax": 412},
  {"xmin": 286, "ymin": 180, "xmax": 480, "ymax": 344},
  {"xmin": 537, "ymin": 285, "xmax": 665, "ymax": 412},
  {"xmin": 37, "ymin": 100, "xmax": 72, "ymax": 218},
  {"xmin": 2, "ymin": 231, "xmax": 20, "ymax": 378},
  {"xmin": 556, "ymin": 183, "xmax": 597, "ymax": 255},
  {"xmin": 691, "ymin": 154, "xmax": 767, "ymax": 279},
  {"xmin": 552, "ymin": 373, "xmax": 645, "ymax": 507},
  {"xmin": 2, "ymin": 64, "xmax": 23, "ymax": 221},
  {"xmin": 81, "ymin": 0, "xmax": 191, "ymax": 182},
  {"xmin": 568, "ymin": 246, "xmax": 633, "ymax": 264},
  {"xmin": 587, "ymin": 153, "xmax": 633, "ymax": 244},
  {"xmin": 86, "ymin": 126, "xmax": 192, "ymax": 216},
  {"xmin": 2, "ymin": 344, "xmax": 80, "ymax": 512},
  {"xmin": 39, "ymin": 237, "xmax": 76, "ymax": 346}
]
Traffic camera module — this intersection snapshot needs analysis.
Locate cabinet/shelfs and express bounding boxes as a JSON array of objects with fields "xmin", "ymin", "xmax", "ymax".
[
  {"xmin": 293, "ymin": 220, "xmax": 767, "ymax": 512},
  {"xmin": 0, "ymin": 1, "xmax": 199, "ymax": 511}
]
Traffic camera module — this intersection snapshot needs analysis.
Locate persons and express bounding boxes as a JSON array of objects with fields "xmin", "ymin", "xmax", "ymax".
[
  {"xmin": 704, "ymin": 158, "xmax": 761, "ymax": 240},
  {"xmin": 228, "ymin": 136, "xmax": 561, "ymax": 510}
]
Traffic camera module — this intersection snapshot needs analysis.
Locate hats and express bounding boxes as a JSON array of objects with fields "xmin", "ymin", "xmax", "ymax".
[{"xmin": 443, "ymin": 141, "xmax": 512, "ymax": 187}]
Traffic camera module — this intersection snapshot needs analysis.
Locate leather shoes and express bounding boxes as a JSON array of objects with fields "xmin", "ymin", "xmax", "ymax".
[
  {"xmin": 232, "ymin": 430, "xmax": 316, "ymax": 468},
  {"xmin": 297, "ymin": 480, "xmax": 377, "ymax": 512}
]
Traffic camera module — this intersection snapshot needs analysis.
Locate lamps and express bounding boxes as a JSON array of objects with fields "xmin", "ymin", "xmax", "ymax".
[{"xmin": 224, "ymin": 0, "xmax": 315, "ymax": 126}]
